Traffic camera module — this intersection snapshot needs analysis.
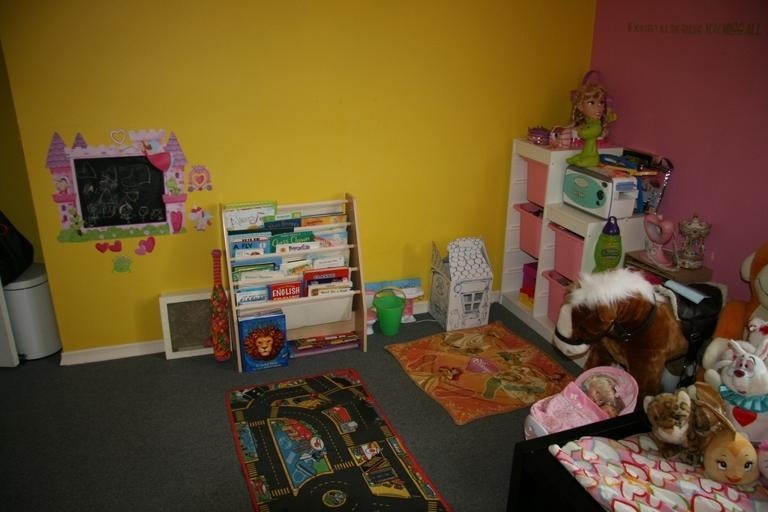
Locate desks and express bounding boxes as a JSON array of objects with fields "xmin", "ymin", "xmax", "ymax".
[{"xmin": 622, "ymin": 240, "xmax": 715, "ymax": 284}]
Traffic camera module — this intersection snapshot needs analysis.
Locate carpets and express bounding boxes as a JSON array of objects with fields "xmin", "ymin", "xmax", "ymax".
[
  {"xmin": 378, "ymin": 316, "xmax": 576, "ymax": 430},
  {"xmin": 222, "ymin": 364, "xmax": 455, "ymax": 511}
]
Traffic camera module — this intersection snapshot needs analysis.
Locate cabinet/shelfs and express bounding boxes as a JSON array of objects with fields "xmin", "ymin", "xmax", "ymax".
[{"xmin": 493, "ymin": 132, "xmax": 661, "ymax": 347}]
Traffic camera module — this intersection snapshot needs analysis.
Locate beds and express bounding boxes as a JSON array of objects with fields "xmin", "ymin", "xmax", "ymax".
[
  {"xmin": 522, "ymin": 362, "xmax": 642, "ymax": 440},
  {"xmin": 502, "ymin": 413, "xmax": 767, "ymax": 512}
]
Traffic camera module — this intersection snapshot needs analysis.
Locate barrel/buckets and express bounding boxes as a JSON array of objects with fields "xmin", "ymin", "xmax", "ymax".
[{"xmin": 372, "ymin": 286, "xmax": 406, "ymax": 336}]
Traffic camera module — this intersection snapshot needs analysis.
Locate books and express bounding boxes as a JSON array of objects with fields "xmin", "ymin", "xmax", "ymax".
[{"xmin": 224, "ymin": 203, "xmax": 352, "ymax": 377}]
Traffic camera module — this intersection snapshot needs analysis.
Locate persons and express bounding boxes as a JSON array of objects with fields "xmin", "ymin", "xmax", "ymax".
[{"xmin": 565, "ymin": 72, "xmax": 609, "ymax": 168}]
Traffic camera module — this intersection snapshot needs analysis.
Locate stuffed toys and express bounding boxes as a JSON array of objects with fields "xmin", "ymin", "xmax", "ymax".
[{"xmin": 524, "ymin": 239, "xmax": 768, "ymax": 487}]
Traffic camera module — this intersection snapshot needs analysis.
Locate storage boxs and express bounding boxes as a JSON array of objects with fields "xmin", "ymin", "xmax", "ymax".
[
  {"xmin": 537, "ymin": 265, "xmax": 579, "ymax": 326},
  {"xmin": 512, "ymin": 200, "xmax": 543, "ymax": 264},
  {"xmin": 544, "ymin": 219, "xmax": 585, "ymax": 284},
  {"xmin": 516, "ymin": 152, "xmax": 550, "ymax": 211}
]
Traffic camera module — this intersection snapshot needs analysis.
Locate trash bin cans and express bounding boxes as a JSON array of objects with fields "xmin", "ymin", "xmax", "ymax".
[{"xmin": 3, "ymin": 262, "xmax": 62, "ymax": 362}]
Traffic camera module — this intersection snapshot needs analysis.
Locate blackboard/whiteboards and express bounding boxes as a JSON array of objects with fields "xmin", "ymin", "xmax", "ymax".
[{"xmin": 73, "ymin": 155, "xmax": 167, "ymax": 228}]
